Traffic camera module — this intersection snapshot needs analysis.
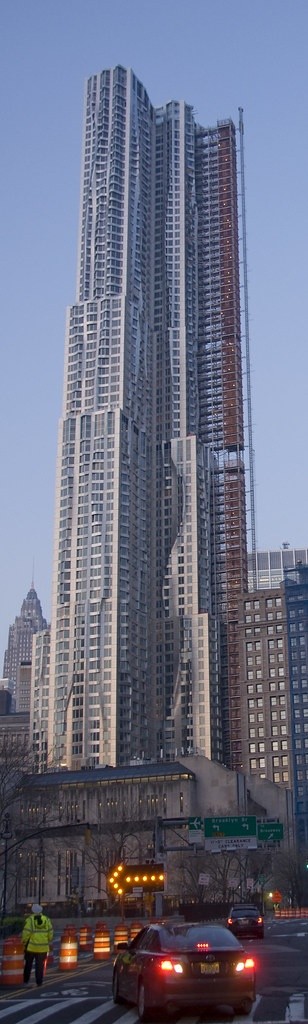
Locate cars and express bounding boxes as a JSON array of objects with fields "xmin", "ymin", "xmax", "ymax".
[{"xmin": 112, "ymin": 922, "xmax": 256, "ymax": 1023}]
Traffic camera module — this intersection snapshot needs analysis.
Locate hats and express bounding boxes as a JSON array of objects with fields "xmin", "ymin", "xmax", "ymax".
[{"xmin": 31, "ymin": 904, "xmax": 44, "ymax": 914}]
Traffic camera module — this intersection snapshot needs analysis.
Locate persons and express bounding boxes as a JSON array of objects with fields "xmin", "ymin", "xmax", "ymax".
[{"xmin": 21, "ymin": 905, "xmax": 53, "ymax": 987}]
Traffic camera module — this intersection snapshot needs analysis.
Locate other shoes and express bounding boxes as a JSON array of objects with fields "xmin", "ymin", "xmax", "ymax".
[
  {"xmin": 22, "ymin": 983, "xmax": 29, "ymax": 987},
  {"xmin": 36, "ymin": 983, "xmax": 42, "ymax": 988}
]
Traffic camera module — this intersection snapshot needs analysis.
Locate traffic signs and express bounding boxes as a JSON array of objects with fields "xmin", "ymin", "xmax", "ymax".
[
  {"xmin": 256, "ymin": 822, "xmax": 284, "ymax": 841},
  {"xmin": 205, "ymin": 817, "xmax": 258, "ymax": 851}
]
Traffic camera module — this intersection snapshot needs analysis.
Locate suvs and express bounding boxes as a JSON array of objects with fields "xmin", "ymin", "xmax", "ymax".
[{"xmin": 227, "ymin": 904, "xmax": 265, "ymax": 939}]
